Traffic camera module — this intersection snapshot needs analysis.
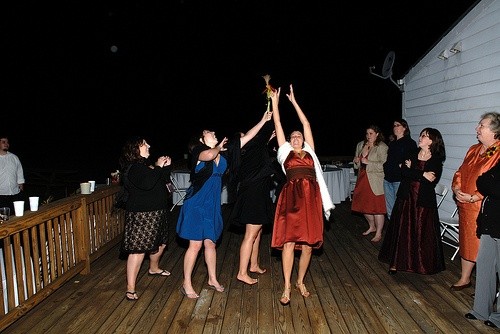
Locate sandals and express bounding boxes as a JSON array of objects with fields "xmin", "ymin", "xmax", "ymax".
[{"xmin": 280, "ymin": 287, "xmax": 291, "ymax": 306}]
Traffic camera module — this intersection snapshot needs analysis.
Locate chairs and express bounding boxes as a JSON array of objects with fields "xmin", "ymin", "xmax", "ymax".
[
  {"xmin": 438, "ymin": 207, "xmax": 460, "ymax": 260},
  {"xmin": 349, "ymin": 176, "xmax": 357, "ymax": 201},
  {"xmin": 435, "ymin": 184, "xmax": 449, "ymax": 207},
  {"xmin": 170, "ymin": 175, "xmax": 188, "ymax": 211}
]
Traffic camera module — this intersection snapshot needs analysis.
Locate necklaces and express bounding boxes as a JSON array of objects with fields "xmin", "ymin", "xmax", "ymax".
[{"xmin": 420, "ymin": 151, "xmax": 428, "ymax": 157}]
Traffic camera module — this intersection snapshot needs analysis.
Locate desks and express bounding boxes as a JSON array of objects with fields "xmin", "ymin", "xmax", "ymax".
[
  {"xmin": 171, "ymin": 170, "xmax": 228, "ymax": 205},
  {"xmin": 322, "ymin": 164, "xmax": 355, "ymax": 204}
]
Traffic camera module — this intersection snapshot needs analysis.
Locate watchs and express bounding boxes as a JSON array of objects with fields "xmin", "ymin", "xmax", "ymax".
[{"xmin": 471, "ymin": 195, "xmax": 474, "ymax": 203}]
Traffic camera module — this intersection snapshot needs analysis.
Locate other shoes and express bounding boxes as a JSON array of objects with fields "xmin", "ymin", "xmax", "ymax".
[{"xmin": 450, "ymin": 280, "xmax": 472, "ymax": 291}]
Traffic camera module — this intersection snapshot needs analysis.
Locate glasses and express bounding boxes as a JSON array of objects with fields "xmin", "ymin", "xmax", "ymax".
[
  {"xmin": 393, "ymin": 124, "xmax": 401, "ymax": 127},
  {"xmin": 421, "ymin": 134, "xmax": 429, "ymax": 138}
]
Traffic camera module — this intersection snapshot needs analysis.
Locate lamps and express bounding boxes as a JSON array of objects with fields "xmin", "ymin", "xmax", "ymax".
[
  {"xmin": 437, "ymin": 49, "xmax": 449, "ymax": 59},
  {"xmin": 449, "ymin": 41, "xmax": 462, "ymax": 53}
]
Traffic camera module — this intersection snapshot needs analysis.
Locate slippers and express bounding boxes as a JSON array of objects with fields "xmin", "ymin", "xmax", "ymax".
[
  {"xmin": 180, "ymin": 286, "xmax": 200, "ymax": 299},
  {"xmin": 125, "ymin": 291, "xmax": 139, "ymax": 301},
  {"xmin": 207, "ymin": 284, "xmax": 225, "ymax": 293},
  {"xmin": 148, "ymin": 269, "xmax": 173, "ymax": 276}
]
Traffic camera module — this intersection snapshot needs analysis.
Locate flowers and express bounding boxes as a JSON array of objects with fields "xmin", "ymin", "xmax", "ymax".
[{"xmin": 262, "ymin": 74, "xmax": 276, "ymax": 113}]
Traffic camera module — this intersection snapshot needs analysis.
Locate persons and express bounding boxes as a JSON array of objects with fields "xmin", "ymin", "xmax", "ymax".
[
  {"xmin": 226, "ymin": 129, "xmax": 285, "ymax": 285},
  {"xmin": 117, "ymin": 137, "xmax": 172, "ymax": 302},
  {"xmin": 465, "ymin": 160, "xmax": 500, "ymax": 328},
  {"xmin": 270, "ymin": 84, "xmax": 336, "ymax": 305},
  {"xmin": 378, "ymin": 127, "xmax": 447, "ymax": 276},
  {"xmin": 450, "ymin": 111, "xmax": 500, "ymax": 292},
  {"xmin": 176, "ymin": 110, "xmax": 272, "ymax": 299},
  {"xmin": 0, "ymin": 137, "xmax": 25, "ymax": 217},
  {"xmin": 351, "ymin": 119, "xmax": 417, "ymax": 242}
]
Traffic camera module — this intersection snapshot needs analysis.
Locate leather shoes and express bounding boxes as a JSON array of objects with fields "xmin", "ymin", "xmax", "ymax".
[
  {"xmin": 465, "ymin": 313, "xmax": 476, "ymax": 319},
  {"xmin": 484, "ymin": 320, "xmax": 495, "ymax": 326}
]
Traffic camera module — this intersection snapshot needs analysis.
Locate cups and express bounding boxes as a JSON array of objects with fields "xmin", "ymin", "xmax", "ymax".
[
  {"xmin": 87, "ymin": 180, "xmax": 95, "ymax": 192},
  {"xmin": 13, "ymin": 201, "xmax": 25, "ymax": 216},
  {"xmin": 80, "ymin": 183, "xmax": 91, "ymax": 194},
  {"xmin": 29, "ymin": 197, "xmax": 40, "ymax": 212},
  {"xmin": 0, "ymin": 206, "xmax": 10, "ymax": 221},
  {"xmin": 107, "ymin": 176, "xmax": 111, "ymax": 185}
]
